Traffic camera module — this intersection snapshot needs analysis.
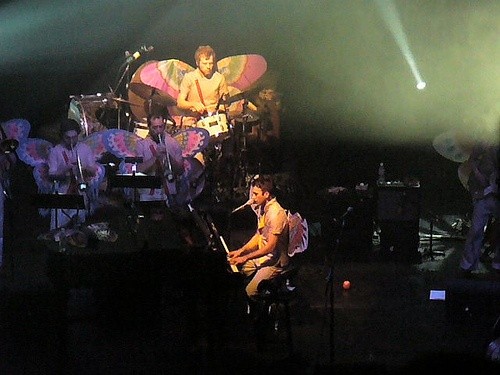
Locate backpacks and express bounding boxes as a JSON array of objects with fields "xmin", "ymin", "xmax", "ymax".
[{"xmin": 287, "ymin": 210, "xmax": 309, "ymax": 258}]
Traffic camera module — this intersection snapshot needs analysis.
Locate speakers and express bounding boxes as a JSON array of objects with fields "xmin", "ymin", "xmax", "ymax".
[{"xmin": 374, "ymin": 185, "xmax": 420, "ymax": 221}]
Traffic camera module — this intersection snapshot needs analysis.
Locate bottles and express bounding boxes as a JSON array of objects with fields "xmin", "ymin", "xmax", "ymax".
[{"xmin": 379, "ymin": 163, "xmax": 384, "ymax": 185}]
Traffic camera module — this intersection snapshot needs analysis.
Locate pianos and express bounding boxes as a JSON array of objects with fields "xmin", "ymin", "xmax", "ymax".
[{"xmin": 40, "ymin": 199, "xmax": 296, "ymax": 353}]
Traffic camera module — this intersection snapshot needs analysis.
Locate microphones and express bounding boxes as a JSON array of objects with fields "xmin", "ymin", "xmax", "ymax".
[
  {"xmin": 215, "ymin": 93, "xmax": 224, "ymax": 109},
  {"xmin": 341, "ymin": 206, "xmax": 353, "ymax": 219},
  {"xmin": 232, "ymin": 199, "xmax": 255, "ymax": 213}
]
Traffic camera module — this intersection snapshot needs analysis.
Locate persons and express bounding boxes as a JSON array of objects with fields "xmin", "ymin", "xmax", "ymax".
[
  {"xmin": 134, "ymin": 111, "xmax": 184, "ymax": 215},
  {"xmin": 229, "ymin": 178, "xmax": 288, "ymax": 296},
  {"xmin": 177, "ymin": 44, "xmax": 231, "ymax": 119},
  {"xmin": 462, "ymin": 134, "xmax": 500, "ymax": 273},
  {"xmin": 46, "ymin": 119, "xmax": 98, "ymax": 232}
]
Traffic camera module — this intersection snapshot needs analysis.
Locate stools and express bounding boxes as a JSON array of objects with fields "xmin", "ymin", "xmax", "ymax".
[{"xmin": 235, "ymin": 253, "xmax": 299, "ymax": 353}]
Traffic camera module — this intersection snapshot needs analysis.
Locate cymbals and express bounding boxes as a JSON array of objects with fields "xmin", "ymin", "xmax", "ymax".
[
  {"xmin": 125, "ymin": 82, "xmax": 180, "ymax": 107},
  {"xmin": 216, "ymin": 85, "xmax": 266, "ymax": 105},
  {"xmin": 112, "ymin": 96, "xmax": 146, "ymax": 109},
  {"xmin": 227, "ymin": 111, "xmax": 272, "ymax": 124}
]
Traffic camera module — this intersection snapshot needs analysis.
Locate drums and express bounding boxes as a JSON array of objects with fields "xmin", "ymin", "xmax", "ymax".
[
  {"xmin": 195, "ymin": 111, "xmax": 234, "ymax": 149},
  {"xmin": 133, "ymin": 120, "xmax": 152, "ymax": 138}
]
